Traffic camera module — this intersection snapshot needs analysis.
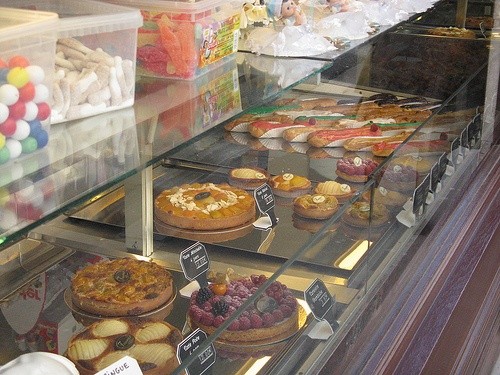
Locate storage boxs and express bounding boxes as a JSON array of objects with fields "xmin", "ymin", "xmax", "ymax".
[
  {"xmin": 134, "ymin": 60, "xmax": 246, "ymax": 156},
  {"xmin": 0, "ymin": 8, "xmax": 61, "ymax": 169},
  {"xmin": 0, "ymin": 0, "xmax": 145, "ymax": 128},
  {"xmin": 0, "ymin": 154, "xmax": 55, "ymax": 239},
  {"xmin": 110, "ymin": 0, "xmax": 241, "ymax": 84},
  {"xmin": 51, "ymin": 105, "xmax": 139, "ymax": 202}
]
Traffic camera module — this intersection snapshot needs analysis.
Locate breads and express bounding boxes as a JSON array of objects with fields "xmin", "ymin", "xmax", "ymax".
[{"xmin": 225, "ymin": 93, "xmax": 483, "ymax": 178}]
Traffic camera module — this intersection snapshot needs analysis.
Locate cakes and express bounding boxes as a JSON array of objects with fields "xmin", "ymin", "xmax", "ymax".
[
  {"xmin": 229, "ymin": 155, "xmax": 418, "ymax": 228},
  {"xmin": 68, "ymin": 256, "xmax": 299, "ymax": 375},
  {"xmin": 153, "ymin": 183, "xmax": 256, "ymax": 232}
]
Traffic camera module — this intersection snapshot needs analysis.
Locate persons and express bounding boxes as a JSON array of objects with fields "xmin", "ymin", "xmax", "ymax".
[
  {"xmin": 327, "ymin": 0, "xmax": 348, "ymax": 13},
  {"xmin": 266, "ymin": 0, "xmax": 302, "ymax": 26}
]
